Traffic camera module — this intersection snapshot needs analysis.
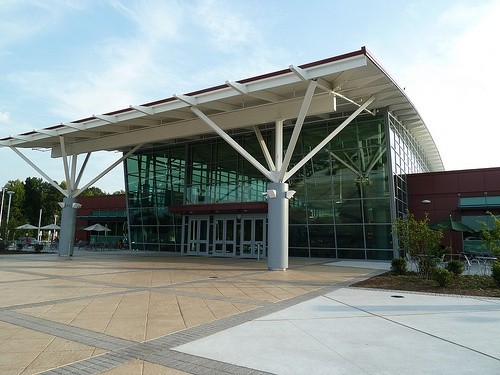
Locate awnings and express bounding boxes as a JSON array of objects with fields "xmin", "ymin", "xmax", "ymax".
[{"xmin": 457, "ymin": 196, "xmax": 499, "ymax": 208}]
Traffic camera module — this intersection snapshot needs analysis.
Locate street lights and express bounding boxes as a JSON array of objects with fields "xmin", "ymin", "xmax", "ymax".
[
  {"xmin": 54, "ymin": 214, "xmax": 59, "ymax": 240},
  {"xmin": 0, "ymin": 187, "xmax": 9, "ymax": 226},
  {"xmin": 6, "ymin": 190, "xmax": 15, "ymax": 232}
]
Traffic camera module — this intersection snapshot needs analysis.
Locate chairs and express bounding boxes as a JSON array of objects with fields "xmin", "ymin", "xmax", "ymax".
[
  {"xmin": 459, "ymin": 253, "xmax": 488, "ymax": 275},
  {"xmin": 406, "ymin": 253, "xmax": 419, "ymax": 272}
]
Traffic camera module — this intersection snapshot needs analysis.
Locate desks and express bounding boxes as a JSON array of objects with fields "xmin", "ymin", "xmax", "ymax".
[{"xmin": 476, "ymin": 256, "xmax": 497, "ymax": 261}]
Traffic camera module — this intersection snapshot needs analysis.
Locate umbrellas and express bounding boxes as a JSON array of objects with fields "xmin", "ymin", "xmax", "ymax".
[
  {"xmin": 428, "ymin": 214, "xmax": 475, "ymax": 261},
  {"xmin": 14, "ymin": 224, "xmax": 38, "ymax": 239},
  {"xmin": 39, "ymin": 223, "xmax": 61, "ymax": 240},
  {"xmin": 82, "ymin": 224, "xmax": 112, "ymax": 243}
]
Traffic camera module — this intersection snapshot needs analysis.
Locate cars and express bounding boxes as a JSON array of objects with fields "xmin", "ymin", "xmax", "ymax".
[{"xmin": 15, "ymin": 236, "xmax": 44, "ymax": 251}]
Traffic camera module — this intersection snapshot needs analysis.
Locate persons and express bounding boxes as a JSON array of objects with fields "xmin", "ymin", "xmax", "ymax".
[{"xmin": 24, "ymin": 235, "xmax": 32, "ymax": 248}]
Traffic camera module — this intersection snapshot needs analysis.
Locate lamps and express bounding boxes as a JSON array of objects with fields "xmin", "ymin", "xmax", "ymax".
[
  {"xmin": 72, "ymin": 202, "xmax": 81, "ymax": 209},
  {"xmin": 262, "ymin": 192, "xmax": 268, "ymax": 200},
  {"xmin": 267, "ymin": 189, "xmax": 277, "ymax": 198},
  {"xmin": 57, "ymin": 202, "xmax": 66, "ymax": 208},
  {"xmin": 286, "ymin": 190, "xmax": 296, "ymax": 200}
]
